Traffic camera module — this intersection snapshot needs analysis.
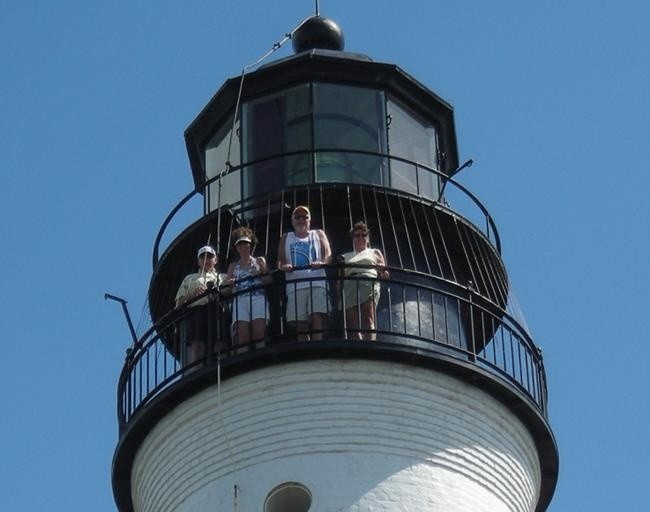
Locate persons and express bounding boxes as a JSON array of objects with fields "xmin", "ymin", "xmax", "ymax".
[
  {"xmin": 219, "ymin": 227, "xmax": 271, "ymax": 355},
  {"xmin": 174, "ymin": 246, "xmax": 227, "ymax": 367},
  {"xmin": 336, "ymin": 220, "xmax": 389, "ymax": 341},
  {"xmin": 277, "ymin": 205, "xmax": 333, "ymax": 342}
]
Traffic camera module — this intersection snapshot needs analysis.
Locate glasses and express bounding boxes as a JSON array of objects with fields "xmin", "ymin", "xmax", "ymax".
[
  {"xmin": 294, "ymin": 215, "xmax": 309, "ymax": 219},
  {"xmin": 353, "ymin": 232, "xmax": 368, "ymax": 237}
]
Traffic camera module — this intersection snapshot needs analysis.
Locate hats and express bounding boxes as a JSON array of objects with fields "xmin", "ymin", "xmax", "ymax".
[
  {"xmin": 197, "ymin": 246, "xmax": 217, "ymax": 259},
  {"xmin": 234, "ymin": 236, "xmax": 252, "ymax": 246},
  {"xmin": 293, "ymin": 205, "xmax": 311, "ymax": 215}
]
